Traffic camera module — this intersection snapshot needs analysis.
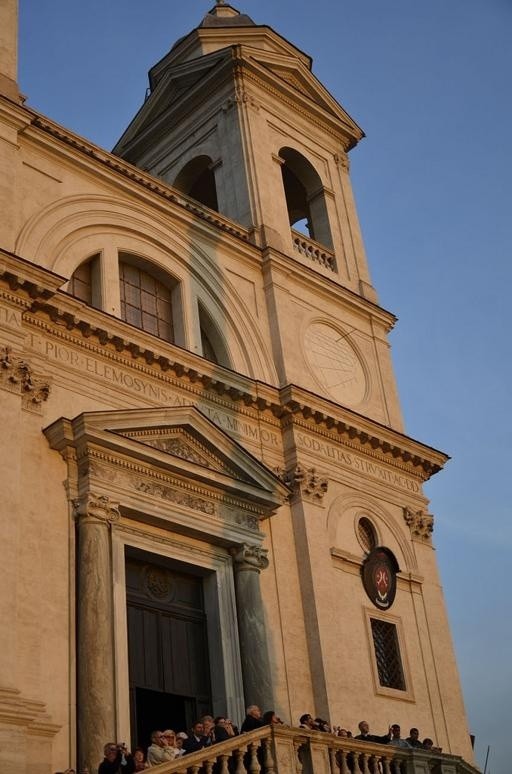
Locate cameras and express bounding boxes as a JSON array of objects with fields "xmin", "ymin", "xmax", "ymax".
[{"xmin": 111, "ymin": 742, "xmax": 126, "ymax": 752}]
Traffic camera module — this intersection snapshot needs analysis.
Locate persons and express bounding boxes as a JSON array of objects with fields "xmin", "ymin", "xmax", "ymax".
[
  {"xmin": 98, "ymin": 716, "xmax": 240, "ymax": 773},
  {"xmin": 65, "ymin": 768, "xmax": 75, "ymax": 773},
  {"xmin": 299, "ymin": 714, "xmax": 393, "ymax": 774},
  {"xmin": 387, "ymin": 725, "xmax": 434, "ymax": 749},
  {"xmin": 240, "ymin": 705, "xmax": 285, "ymax": 773}
]
